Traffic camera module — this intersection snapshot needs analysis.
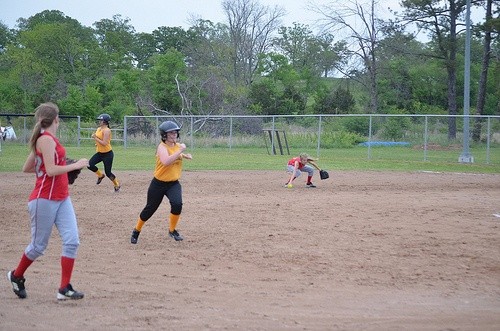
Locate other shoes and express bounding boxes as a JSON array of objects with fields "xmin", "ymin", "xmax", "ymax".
[
  {"xmin": 169, "ymin": 229, "xmax": 183, "ymax": 242},
  {"xmin": 284, "ymin": 183, "xmax": 288, "ymax": 188},
  {"xmin": 113, "ymin": 183, "xmax": 121, "ymax": 193},
  {"xmin": 130, "ymin": 228, "xmax": 141, "ymax": 244},
  {"xmin": 305, "ymin": 182, "xmax": 316, "ymax": 188},
  {"xmin": 96, "ymin": 173, "xmax": 106, "ymax": 184}
]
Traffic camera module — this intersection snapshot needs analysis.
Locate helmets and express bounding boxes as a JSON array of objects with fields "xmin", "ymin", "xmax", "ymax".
[
  {"xmin": 97, "ymin": 114, "xmax": 111, "ymax": 123},
  {"xmin": 159, "ymin": 121, "xmax": 181, "ymax": 134}
]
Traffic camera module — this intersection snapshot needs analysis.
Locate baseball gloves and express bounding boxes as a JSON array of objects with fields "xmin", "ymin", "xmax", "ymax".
[
  {"xmin": 67, "ymin": 158, "xmax": 81, "ymax": 183},
  {"xmin": 320, "ymin": 169, "xmax": 329, "ymax": 179}
]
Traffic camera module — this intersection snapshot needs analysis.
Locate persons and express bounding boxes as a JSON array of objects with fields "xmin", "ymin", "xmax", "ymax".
[
  {"xmin": 283, "ymin": 153, "xmax": 329, "ymax": 188},
  {"xmin": 87, "ymin": 114, "xmax": 120, "ymax": 191},
  {"xmin": 7, "ymin": 101, "xmax": 90, "ymax": 300},
  {"xmin": 131, "ymin": 121, "xmax": 192, "ymax": 244}
]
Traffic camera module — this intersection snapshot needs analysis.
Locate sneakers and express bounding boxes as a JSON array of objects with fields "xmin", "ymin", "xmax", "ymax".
[
  {"xmin": 57, "ymin": 284, "xmax": 84, "ymax": 301},
  {"xmin": 7, "ymin": 270, "xmax": 28, "ymax": 299}
]
